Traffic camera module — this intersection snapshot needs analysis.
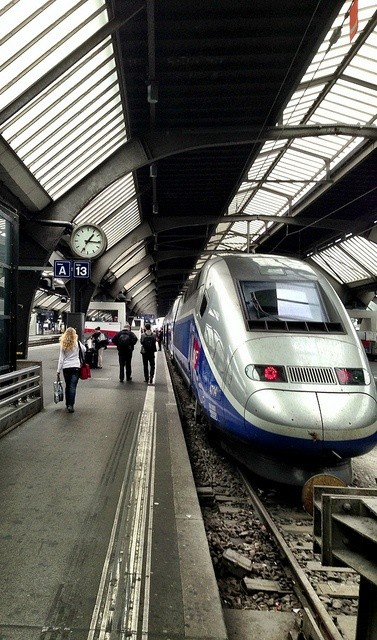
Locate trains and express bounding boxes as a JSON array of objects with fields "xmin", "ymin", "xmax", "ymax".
[{"xmin": 161, "ymin": 252, "xmax": 376, "ymax": 505}]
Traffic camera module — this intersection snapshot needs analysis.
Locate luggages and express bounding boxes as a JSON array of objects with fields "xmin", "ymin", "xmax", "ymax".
[{"xmin": 84, "ymin": 343, "xmax": 98, "ymax": 369}]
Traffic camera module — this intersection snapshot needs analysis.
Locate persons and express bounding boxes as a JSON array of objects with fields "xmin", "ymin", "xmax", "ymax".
[
  {"xmin": 56, "ymin": 326, "xmax": 86, "ymax": 413},
  {"xmin": 111, "ymin": 325, "xmax": 137, "ymax": 382},
  {"xmin": 139, "ymin": 324, "xmax": 156, "ymax": 383},
  {"xmin": 152, "ymin": 328, "xmax": 163, "ymax": 351},
  {"xmin": 90, "ymin": 326, "xmax": 108, "ymax": 368}
]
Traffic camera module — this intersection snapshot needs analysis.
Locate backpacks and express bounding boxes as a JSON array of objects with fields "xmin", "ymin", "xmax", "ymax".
[
  {"xmin": 97, "ymin": 332, "xmax": 107, "ymax": 343},
  {"xmin": 141, "ymin": 333, "xmax": 157, "ymax": 352},
  {"xmin": 117, "ymin": 331, "xmax": 134, "ymax": 351}
]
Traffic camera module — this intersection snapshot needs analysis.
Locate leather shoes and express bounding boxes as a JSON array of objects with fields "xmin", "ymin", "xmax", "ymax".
[{"xmin": 67, "ymin": 407, "xmax": 74, "ymax": 413}]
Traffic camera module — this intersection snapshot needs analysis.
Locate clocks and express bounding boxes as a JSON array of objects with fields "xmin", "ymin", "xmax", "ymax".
[{"xmin": 70, "ymin": 223, "xmax": 108, "ymax": 261}]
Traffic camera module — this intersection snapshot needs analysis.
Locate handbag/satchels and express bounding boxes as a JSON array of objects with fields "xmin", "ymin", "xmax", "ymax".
[
  {"xmin": 78, "ymin": 341, "xmax": 91, "ymax": 380},
  {"xmin": 53, "ymin": 376, "xmax": 63, "ymax": 404}
]
